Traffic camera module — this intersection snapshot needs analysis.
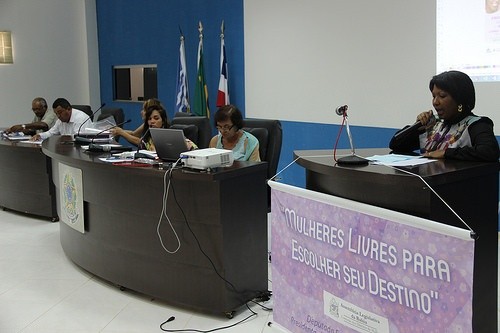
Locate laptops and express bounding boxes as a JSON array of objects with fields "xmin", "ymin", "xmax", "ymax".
[{"xmin": 149, "ymin": 129, "xmax": 188, "ymax": 162}]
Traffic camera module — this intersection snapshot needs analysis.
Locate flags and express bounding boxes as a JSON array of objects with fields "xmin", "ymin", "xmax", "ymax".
[
  {"xmin": 192, "ymin": 40, "xmax": 210, "ymax": 119},
  {"xmin": 216, "ymin": 38, "xmax": 231, "ymax": 107},
  {"xmin": 176, "ymin": 41, "xmax": 191, "ymax": 113}
]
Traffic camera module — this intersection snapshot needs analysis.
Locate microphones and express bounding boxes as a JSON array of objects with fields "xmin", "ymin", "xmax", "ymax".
[
  {"xmin": 89, "ymin": 120, "xmax": 131, "ymax": 152},
  {"xmin": 76, "ymin": 103, "xmax": 107, "ymax": 145},
  {"xmin": 134, "ymin": 125, "xmax": 155, "ymax": 160},
  {"xmin": 336, "ymin": 105, "xmax": 348, "ymax": 116},
  {"xmin": 396, "ymin": 115, "xmax": 435, "ymax": 140}
]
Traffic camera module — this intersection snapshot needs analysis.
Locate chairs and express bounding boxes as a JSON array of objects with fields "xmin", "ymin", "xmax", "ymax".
[
  {"xmin": 168, "ymin": 112, "xmax": 284, "ymax": 181},
  {"xmin": 71, "ymin": 104, "xmax": 126, "ymax": 144}
]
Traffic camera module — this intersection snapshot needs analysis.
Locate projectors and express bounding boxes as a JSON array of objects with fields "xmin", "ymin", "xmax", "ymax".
[{"xmin": 180, "ymin": 147, "xmax": 234, "ymax": 172}]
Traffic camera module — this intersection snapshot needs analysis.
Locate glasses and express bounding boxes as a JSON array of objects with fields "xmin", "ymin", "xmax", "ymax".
[{"xmin": 216, "ymin": 124, "xmax": 235, "ymax": 132}]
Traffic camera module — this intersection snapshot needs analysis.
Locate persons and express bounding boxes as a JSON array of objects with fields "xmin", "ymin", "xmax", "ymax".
[
  {"xmin": 109, "ymin": 100, "xmax": 160, "ymax": 147},
  {"xmin": 389, "ymin": 71, "xmax": 500, "ymax": 162},
  {"xmin": 209, "ymin": 105, "xmax": 261, "ymax": 161},
  {"xmin": 30, "ymin": 98, "xmax": 93, "ymax": 142},
  {"xmin": 4, "ymin": 97, "xmax": 59, "ymax": 142},
  {"xmin": 143, "ymin": 104, "xmax": 198, "ymax": 152}
]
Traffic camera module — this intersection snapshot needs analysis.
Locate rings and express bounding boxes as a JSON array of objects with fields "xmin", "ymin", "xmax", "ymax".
[{"xmin": 428, "ymin": 152, "xmax": 430, "ymax": 156}]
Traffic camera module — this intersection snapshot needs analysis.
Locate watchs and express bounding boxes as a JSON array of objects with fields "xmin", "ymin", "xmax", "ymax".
[{"xmin": 22, "ymin": 124, "xmax": 26, "ymax": 130}]
